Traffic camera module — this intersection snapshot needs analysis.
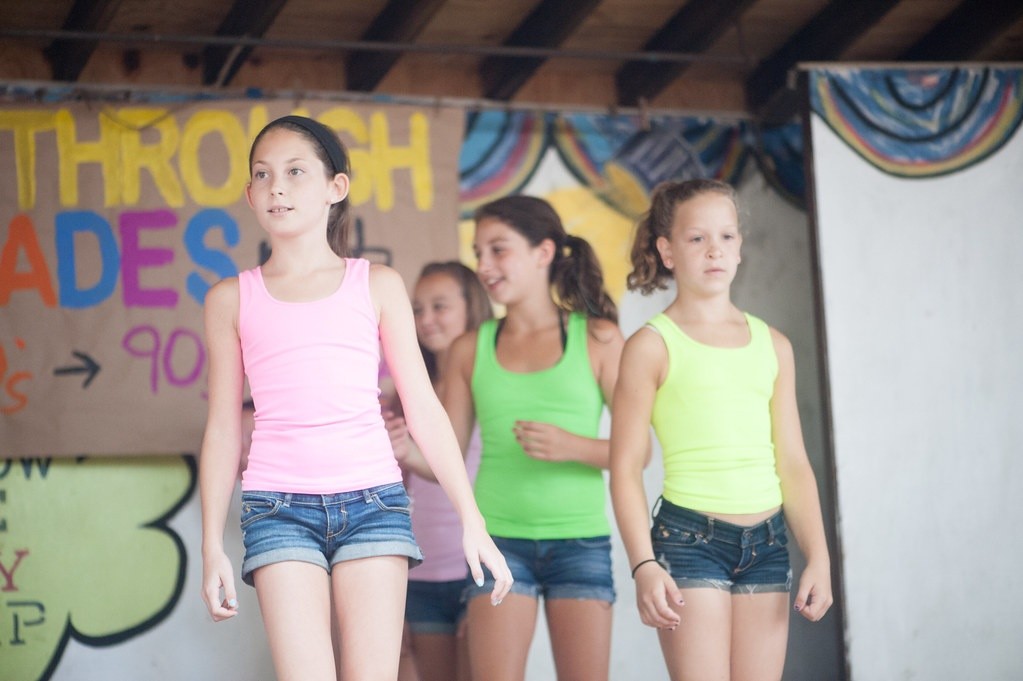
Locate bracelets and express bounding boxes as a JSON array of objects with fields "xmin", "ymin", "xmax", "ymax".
[{"xmin": 631, "ymin": 559, "xmax": 657, "ymax": 578}]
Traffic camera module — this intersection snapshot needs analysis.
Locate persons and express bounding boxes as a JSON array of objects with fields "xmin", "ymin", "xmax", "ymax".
[
  {"xmin": 387, "ymin": 261, "xmax": 498, "ymax": 681},
  {"xmin": 197, "ymin": 113, "xmax": 516, "ymax": 681},
  {"xmin": 380, "ymin": 193, "xmax": 653, "ymax": 681},
  {"xmin": 608, "ymin": 178, "xmax": 834, "ymax": 681}
]
